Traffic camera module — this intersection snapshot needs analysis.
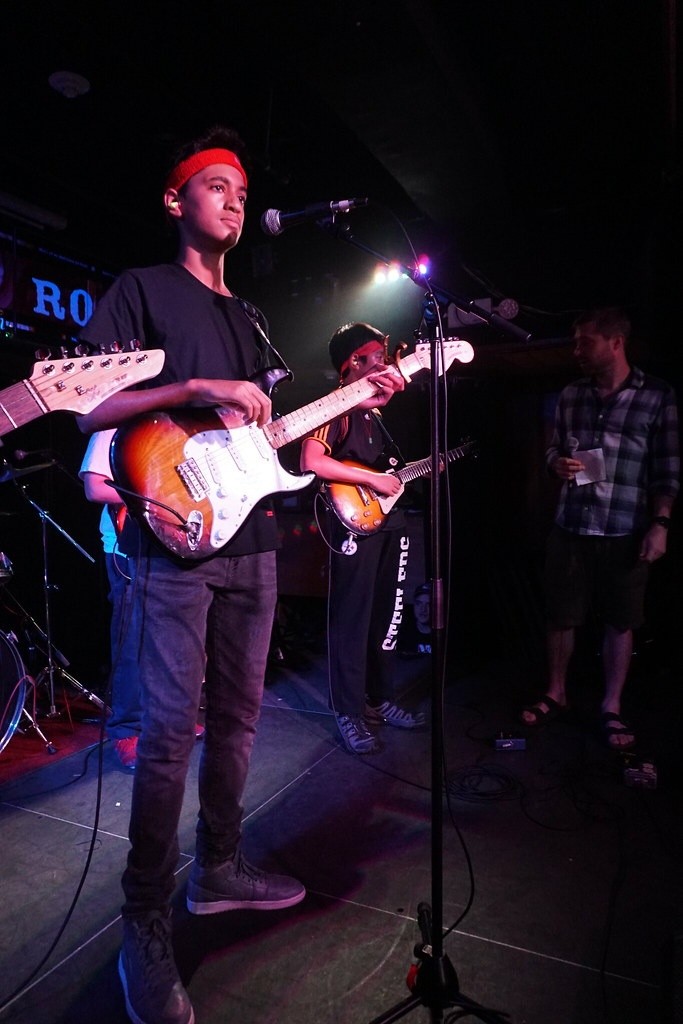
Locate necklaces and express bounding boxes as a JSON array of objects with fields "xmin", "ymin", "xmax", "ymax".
[{"xmin": 359, "ymin": 409, "xmax": 372, "ymax": 444}]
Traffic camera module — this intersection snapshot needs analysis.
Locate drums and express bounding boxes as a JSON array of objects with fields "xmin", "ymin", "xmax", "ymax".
[
  {"xmin": 0, "ymin": 546, "xmax": 15, "ymax": 586},
  {"xmin": 0, "ymin": 626, "xmax": 27, "ymax": 754}
]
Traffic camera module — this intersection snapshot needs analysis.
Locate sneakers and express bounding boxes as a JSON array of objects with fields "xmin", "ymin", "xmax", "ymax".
[
  {"xmin": 336, "ymin": 714, "xmax": 378, "ymax": 754},
  {"xmin": 118, "ymin": 906, "xmax": 196, "ymax": 1024},
  {"xmin": 186, "ymin": 847, "xmax": 306, "ymax": 915},
  {"xmin": 362, "ymin": 699, "xmax": 426, "ymax": 730}
]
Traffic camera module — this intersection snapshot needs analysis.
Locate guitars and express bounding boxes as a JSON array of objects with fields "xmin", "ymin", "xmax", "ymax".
[
  {"xmin": 318, "ymin": 434, "xmax": 478, "ymax": 540},
  {"xmin": 1, "ymin": 336, "xmax": 168, "ymax": 443},
  {"xmin": 108, "ymin": 335, "xmax": 475, "ymax": 568}
]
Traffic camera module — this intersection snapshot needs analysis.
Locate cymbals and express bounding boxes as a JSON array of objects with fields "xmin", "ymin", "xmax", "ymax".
[{"xmin": 0, "ymin": 461, "xmax": 59, "ymax": 483}]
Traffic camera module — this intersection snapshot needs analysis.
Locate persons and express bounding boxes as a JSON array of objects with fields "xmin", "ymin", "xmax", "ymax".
[
  {"xmin": 79, "ymin": 428, "xmax": 206, "ymax": 768},
  {"xmin": 74, "ymin": 126, "xmax": 405, "ymax": 1024},
  {"xmin": 299, "ymin": 321, "xmax": 445, "ymax": 752},
  {"xmin": 519, "ymin": 303, "xmax": 683, "ymax": 751}
]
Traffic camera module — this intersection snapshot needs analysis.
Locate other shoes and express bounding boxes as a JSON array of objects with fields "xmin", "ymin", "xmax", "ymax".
[
  {"xmin": 115, "ymin": 735, "xmax": 140, "ymax": 767},
  {"xmin": 195, "ymin": 720, "xmax": 206, "ymax": 738}
]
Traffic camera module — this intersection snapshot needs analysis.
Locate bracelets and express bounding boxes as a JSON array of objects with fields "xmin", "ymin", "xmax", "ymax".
[{"xmin": 652, "ymin": 515, "xmax": 671, "ymax": 529}]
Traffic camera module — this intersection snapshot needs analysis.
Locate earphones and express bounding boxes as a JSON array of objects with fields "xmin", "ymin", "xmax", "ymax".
[
  {"xmin": 353, "ymin": 361, "xmax": 357, "ymax": 365},
  {"xmin": 169, "ymin": 202, "xmax": 177, "ymax": 209}
]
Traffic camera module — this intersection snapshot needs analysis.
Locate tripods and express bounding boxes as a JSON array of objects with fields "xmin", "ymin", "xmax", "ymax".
[
  {"xmin": 313, "ymin": 218, "xmax": 532, "ymax": 1024},
  {"xmin": 9, "ymin": 478, "xmax": 115, "ymax": 723}
]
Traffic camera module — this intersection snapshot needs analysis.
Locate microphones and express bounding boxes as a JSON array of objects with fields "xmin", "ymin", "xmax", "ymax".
[
  {"xmin": 263, "ymin": 196, "xmax": 369, "ymax": 237},
  {"xmin": 17, "ymin": 449, "xmax": 51, "ymax": 460},
  {"xmin": 564, "ymin": 437, "xmax": 579, "ymax": 486}
]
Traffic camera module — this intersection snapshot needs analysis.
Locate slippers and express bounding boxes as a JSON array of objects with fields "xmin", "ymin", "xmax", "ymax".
[
  {"xmin": 518, "ymin": 693, "xmax": 570, "ymax": 727},
  {"xmin": 598, "ymin": 712, "xmax": 638, "ymax": 748}
]
